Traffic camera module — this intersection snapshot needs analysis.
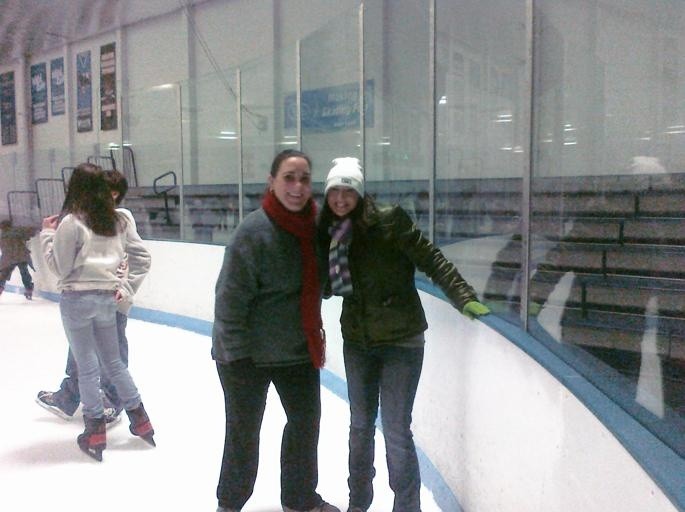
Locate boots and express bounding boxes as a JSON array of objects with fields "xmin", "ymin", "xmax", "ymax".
[
  {"xmin": 0, "ymin": 285, "xmax": 4, "ymax": 295},
  {"xmin": 75, "ymin": 414, "xmax": 108, "ymax": 451},
  {"xmin": 23, "ymin": 283, "xmax": 34, "ymax": 296},
  {"xmin": 124, "ymin": 401, "xmax": 156, "ymax": 438}
]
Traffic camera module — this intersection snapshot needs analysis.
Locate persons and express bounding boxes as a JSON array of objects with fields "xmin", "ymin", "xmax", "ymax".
[
  {"xmin": 314, "ymin": 154, "xmax": 492, "ymax": 511},
  {"xmin": 207, "ymin": 148, "xmax": 340, "ymax": 511},
  {"xmin": 0, "ymin": 217, "xmax": 41, "ymax": 300}
]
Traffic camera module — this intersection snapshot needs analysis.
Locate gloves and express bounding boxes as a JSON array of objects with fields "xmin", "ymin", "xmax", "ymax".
[
  {"xmin": 488, "ymin": 299, "xmax": 543, "ymax": 319},
  {"xmin": 223, "ymin": 356, "xmax": 259, "ymax": 395},
  {"xmin": 460, "ymin": 300, "xmax": 492, "ymax": 322}
]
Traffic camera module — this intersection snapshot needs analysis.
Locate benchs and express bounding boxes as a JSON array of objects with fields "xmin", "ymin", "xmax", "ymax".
[{"xmin": 119, "ymin": 171, "xmax": 685, "ymax": 381}]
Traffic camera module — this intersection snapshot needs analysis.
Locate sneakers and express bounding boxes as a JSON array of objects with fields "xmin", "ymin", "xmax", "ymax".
[
  {"xmin": 100, "ymin": 403, "xmax": 124, "ymax": 425},
  {"xmin": 37, "ymin": 385, "xmax": 82, "ymax": 416},
  {"xmin": 213, "ymin": 492, "xmax": 369, "ymax": 512}
]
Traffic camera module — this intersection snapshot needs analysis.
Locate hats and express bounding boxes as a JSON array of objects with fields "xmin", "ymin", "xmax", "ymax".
[
  {"xmin": 626, "ymin": 155, "xmax": 673, "ymax": 191},
  {"xmin": 320, "ymin": 156, "xmax": 366, "ymax": 201}
]
